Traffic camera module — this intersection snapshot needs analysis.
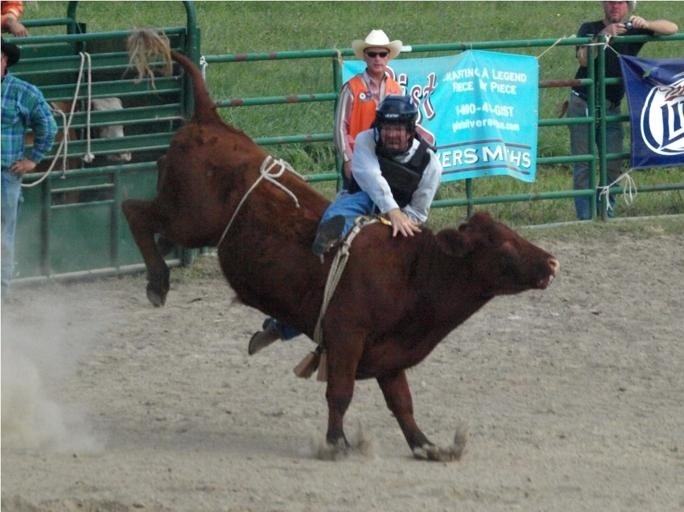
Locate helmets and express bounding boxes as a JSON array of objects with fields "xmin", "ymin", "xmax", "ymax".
[{"xmin": 374, "ymin": 95, "xmax": 418, "ymax": 152}]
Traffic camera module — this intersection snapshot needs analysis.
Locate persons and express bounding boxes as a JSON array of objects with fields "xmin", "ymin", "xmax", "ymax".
[
  {"xmin": 0, "ymin": 31, "xmax": 60, "ymax": 309},
  {"xmin": 328, "ymin": 28, "xmax": 408, "ymax": 202},
  {"xmin": 247, "ymin": 93, "xmax": 443, "ymax": 353},
  {"xmin": 0, "ymin": 0, "xmax": 28, "ymax": 44},
  {"xmin": 564, "ymin": 1, "xmax": 680, "ymax": 225}
]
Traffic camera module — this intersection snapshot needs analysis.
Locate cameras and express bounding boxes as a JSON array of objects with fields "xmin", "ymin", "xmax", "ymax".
[{"xmin": 624, "ymin": 20, "xmax": 633, "ymax": 31}]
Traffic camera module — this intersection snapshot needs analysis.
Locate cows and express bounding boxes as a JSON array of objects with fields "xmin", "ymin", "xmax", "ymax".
[
  {"xmin": 119, "ymin": 25, "xmax": 561, "ymax": 462},
  {"xmin": 22, "ymin": 97, "xmax": 133, "ymax": 206}
]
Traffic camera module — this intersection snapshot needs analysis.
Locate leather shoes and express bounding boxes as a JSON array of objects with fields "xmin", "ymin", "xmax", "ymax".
[
  {"xmin": 313, "ymin": 216, "xmax": 344, "ymax": 255},
  {"xmin": 248, "ymin": 318, "xmax": 278, "ymax": 355}
]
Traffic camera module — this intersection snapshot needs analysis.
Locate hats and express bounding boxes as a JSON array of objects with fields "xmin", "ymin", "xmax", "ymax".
[
  {"xmin": 1, "ymin": 37, "xmax": 19, "ymax": 67},
  {"xmin": 353, "ymin": 29, "xmax": 403, "ymax": 59}
]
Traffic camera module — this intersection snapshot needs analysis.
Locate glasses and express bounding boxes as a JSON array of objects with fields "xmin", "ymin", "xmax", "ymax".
[{"xmin": 368, "ymin": 52, "xmax": 388, "ymax": 57}]
